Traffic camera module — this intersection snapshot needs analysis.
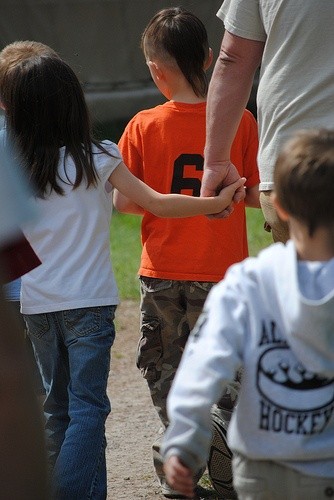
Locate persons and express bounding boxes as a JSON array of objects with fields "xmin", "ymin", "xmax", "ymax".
[
  {"xmin": 111, "ymin": 8, "xmax": 264, "ymax": 500},
  {"xmin": 0, "ymin": 39, "xmax": 248, "ymax": 500},
  {"xmin": 201, "ymin": 0, "xmax": 333, "ymax": 243},
  {"xmin": 160, "ymin": 125, "xmax": 333, "ymax": 500}
]
